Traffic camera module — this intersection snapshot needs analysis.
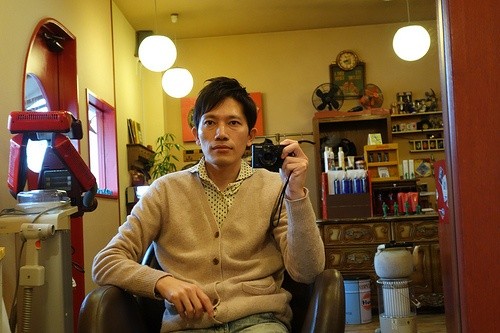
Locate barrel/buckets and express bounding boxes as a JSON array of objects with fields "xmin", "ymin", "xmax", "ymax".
[{"xmin": 341, "ymin": 275, "xmax": 373, "ymax": 325}]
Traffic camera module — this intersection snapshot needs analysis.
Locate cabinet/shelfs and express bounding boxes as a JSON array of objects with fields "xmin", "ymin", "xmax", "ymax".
[
  {"xmin": 390, "ymin": 110, "xmax": 445, "ymax": 152},
  {"xmin": 313, "ymin": 110, "xmax": 394, "ymax": 221},
  {"xmin": 364, "ymin": 143, "xmax": 420, "ymax": 216},
  {"xmin": 315, "ymin": 215, "xmax": 446, "ymax": 311}
]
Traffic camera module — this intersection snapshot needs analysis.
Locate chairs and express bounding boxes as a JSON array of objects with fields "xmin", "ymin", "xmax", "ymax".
[{"xmin": 77, "ymin": 241, "xmax": 346, "ymax": 333}]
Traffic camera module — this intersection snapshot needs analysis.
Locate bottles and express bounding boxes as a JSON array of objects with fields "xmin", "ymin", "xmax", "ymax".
[
  {"xmin": 337, "ymin": 147, "xmax": 345, "ymax": 170},
  {"xmin": 377, "ymin": 194, "xmax": 383, "ymax": 216},
  {"xmin": 352, "ymin": 175, "xmax": 368, "ymax": 194},
  {"xmin": 340, "ymin": 176, "xmax": 352, "ymax": 194},
  {"xmin": 324, "ymin": 146, "xmax": 335, "ymax": 173},
  {"xmin": 385, "ymin": 193, "xmax": 395, "ymax": 215},
  {"xmin": 334, "ymin": 179, "xmax": 340, "ymax": 194}
]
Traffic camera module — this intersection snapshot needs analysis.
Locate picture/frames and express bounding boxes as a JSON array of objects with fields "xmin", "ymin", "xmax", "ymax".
[
  {"xmin": 329, "ymin": 61, "xmax": 364, "ymax": 97},
  {"xmin": 409, "ymin": 138, "xmax": 444, "ymax": 151}
]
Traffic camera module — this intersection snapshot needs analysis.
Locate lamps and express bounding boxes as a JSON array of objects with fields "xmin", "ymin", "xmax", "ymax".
[
  {"xmin": 393, "ymin": 0, "xmax": 431, "ymax": 63},
  {"xmin": 161, "ymin": 12, "xmax": 194, "ymax": 98},
  {"xmin": 139, "ymin": 0, "xmax": 178, "ymax": 73}
]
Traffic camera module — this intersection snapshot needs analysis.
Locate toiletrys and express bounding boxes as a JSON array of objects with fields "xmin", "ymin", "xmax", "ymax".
[
  {"xmin": 347, "ymin": 155, "xmax": 355, "ymax": 170},
  {"xmin": 333, "ymin": 172, "xmax": 367, "ymax": 194},
  {"xmin": 323, "ymin": 146, "xmax": 335, "ymax": 174},
  {"xmin": 338, "ymin": 146, "xmax": 345, "ymax": 172},
  {"xmin": 376, "ymin": 191, "xmax": 419, "ymax": 217}
]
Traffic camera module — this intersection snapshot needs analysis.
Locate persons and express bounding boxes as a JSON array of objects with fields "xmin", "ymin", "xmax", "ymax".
[{"xmin": 92, "ymin": 75, "xmax": 325, "ymax": 333}]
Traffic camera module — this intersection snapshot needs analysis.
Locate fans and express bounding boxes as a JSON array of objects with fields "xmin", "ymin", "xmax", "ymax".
[
  {"xmin": 311, "ymin": 81, "xmax": 345, "ymax": 113},
  {"xmin": 360, "ymin": 83, "xmax": 383, "ymax": 109}
]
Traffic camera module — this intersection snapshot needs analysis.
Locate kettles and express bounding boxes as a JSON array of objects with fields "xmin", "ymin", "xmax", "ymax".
[{"xmin": 373, "ymin": 240, "xmax": 421, "ymax": 279}]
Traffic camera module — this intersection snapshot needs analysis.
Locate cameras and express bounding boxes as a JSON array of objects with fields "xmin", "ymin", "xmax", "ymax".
[{"xmin": 251, "ymin": 144, "xmax": 292, "ymax": 172}]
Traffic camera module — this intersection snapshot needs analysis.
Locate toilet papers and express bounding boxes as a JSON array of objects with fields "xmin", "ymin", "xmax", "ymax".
[{"xmin": 20, "ymin": 223, "xmax": 56, "ymax": 237}]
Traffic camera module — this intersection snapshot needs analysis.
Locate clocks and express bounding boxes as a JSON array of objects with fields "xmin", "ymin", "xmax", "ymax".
[{"xmin": 336, "ymin": 48, "xmax": 359, "ymax": 70}]
